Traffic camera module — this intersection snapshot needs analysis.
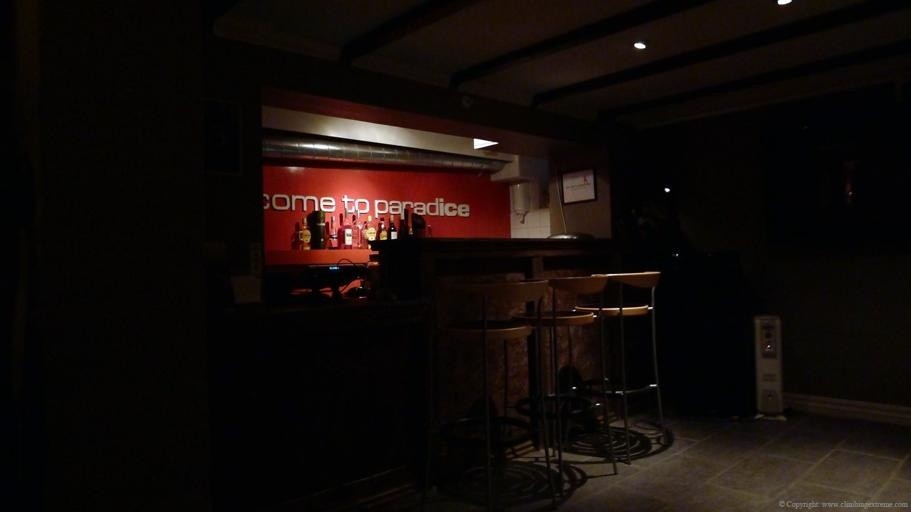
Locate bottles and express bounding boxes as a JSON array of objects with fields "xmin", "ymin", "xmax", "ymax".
[{"xmin": 298, "ymin": 203, "xmax": 413, "ymax": 251}]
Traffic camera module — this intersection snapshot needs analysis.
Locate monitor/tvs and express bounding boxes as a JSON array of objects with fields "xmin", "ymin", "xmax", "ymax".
[{"xmin": 297, "ymin": 263, "xmax": 363, "ymax": 289}]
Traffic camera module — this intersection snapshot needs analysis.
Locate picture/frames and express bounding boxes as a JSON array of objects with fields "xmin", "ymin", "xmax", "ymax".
[
  {"xmin": 201, "ymin": 96, "xmax": 245, "ymax": 177},
  {"xmin": 559, "ymin": 167, "xmax": 599, "ymax": 204}
]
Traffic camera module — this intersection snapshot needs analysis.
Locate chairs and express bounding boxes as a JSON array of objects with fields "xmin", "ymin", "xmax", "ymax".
[
  {"xmin": 433, "ymin": 276, "xmax": 558, "ymax": 512},
  {"xmin": 505, "ymin": 275, "xmax": 622, "ymax": 484},
  {"xmin": 587, "ymin": 272, "xmax": 666, "ymax": 478}
]
